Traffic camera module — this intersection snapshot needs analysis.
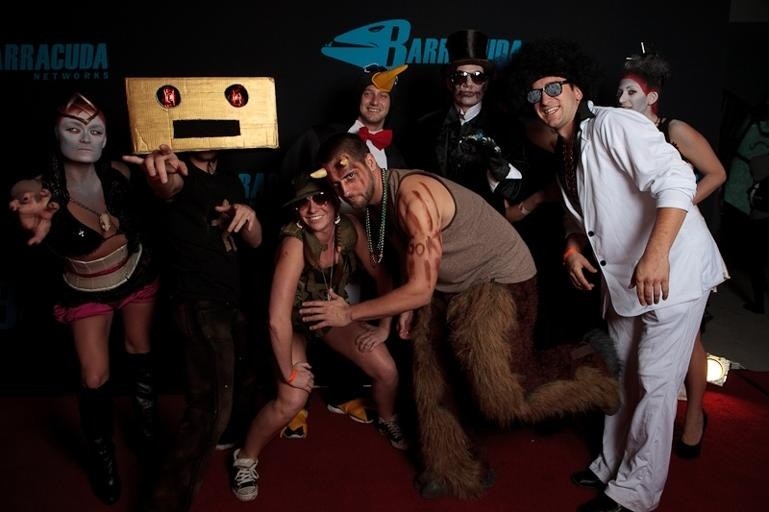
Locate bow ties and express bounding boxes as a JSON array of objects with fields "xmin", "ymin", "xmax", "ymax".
[{"xmin": 359, "ymin": 127, "xmax": 393, "ymax": 150}]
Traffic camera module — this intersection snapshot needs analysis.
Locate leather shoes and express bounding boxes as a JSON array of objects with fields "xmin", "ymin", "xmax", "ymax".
[
  {"xmin": 571, "ymin": 469, "xmax": 604, "ymax": 486},
  {"xmin": 583, "ymin": 493, "xmax": 632, "ymax": 511}
]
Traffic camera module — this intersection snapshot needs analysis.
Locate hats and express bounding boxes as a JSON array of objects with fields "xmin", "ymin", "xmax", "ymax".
[
  {"xmin": 281, "ymin": 176, "xmax": 334, "ymax": 204},
  {"xmin": 445, "ymin": 29, "xmax": 492, "ymax": 68},
  {"xmin": 371, "ymin": 64, "xmax": 408, "ymax": 92},
  {"xmin": 527, "ymin": 53, "xmax": 575, "ymax": 87}
]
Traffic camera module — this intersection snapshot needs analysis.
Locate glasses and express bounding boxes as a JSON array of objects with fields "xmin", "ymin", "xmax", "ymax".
[
  {"xmin": 449, "ymin": 70, "xmax": 487, "ymax": 85},
  {"xmin": 527, "ymin": 79, "xmax": 572, "ymax": 104},
  {"xmin": 294, "ymin": 192, "xmax": 330, "ymax": 209}
]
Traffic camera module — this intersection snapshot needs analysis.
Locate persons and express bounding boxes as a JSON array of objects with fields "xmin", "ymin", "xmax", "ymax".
[{"xmin": 10, "ymin": 29, "xmax": 730, "ymax": 510}]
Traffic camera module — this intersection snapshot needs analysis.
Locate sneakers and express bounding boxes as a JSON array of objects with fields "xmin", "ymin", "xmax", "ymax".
[
  {"xmin": 328, "ymin": 397, "xmax": 374, "ymax": 424},
  {"xmin": 231, "ymin": 448, "xmax": 259, "ymax": 500},
  {"xmin": 281, "ymin": 410, "xmax": 308, "ymax": 439},
  {"xmin": 379, "ymin": 414, "xmax": 408, "ymax": 449}
]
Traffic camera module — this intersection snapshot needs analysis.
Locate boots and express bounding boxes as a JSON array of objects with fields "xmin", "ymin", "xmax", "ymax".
[
  {"xmin": 121, "ymin": 352, "xmax": 159, "ymax": 453},
  {"xmin": 87, "ymin": 380, "xmax": 117, "ymax": 493}
]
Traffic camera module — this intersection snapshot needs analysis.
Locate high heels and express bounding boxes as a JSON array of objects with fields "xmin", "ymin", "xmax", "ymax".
[{"xmin": 680, "ymin": 408, "xmax": 707, "ymax": 459}]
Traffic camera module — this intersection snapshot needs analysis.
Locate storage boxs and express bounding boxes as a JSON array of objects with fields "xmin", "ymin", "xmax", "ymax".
[{"xmin": 122, "ymin": 75, "xmax": 282, "ymax": 157}]
